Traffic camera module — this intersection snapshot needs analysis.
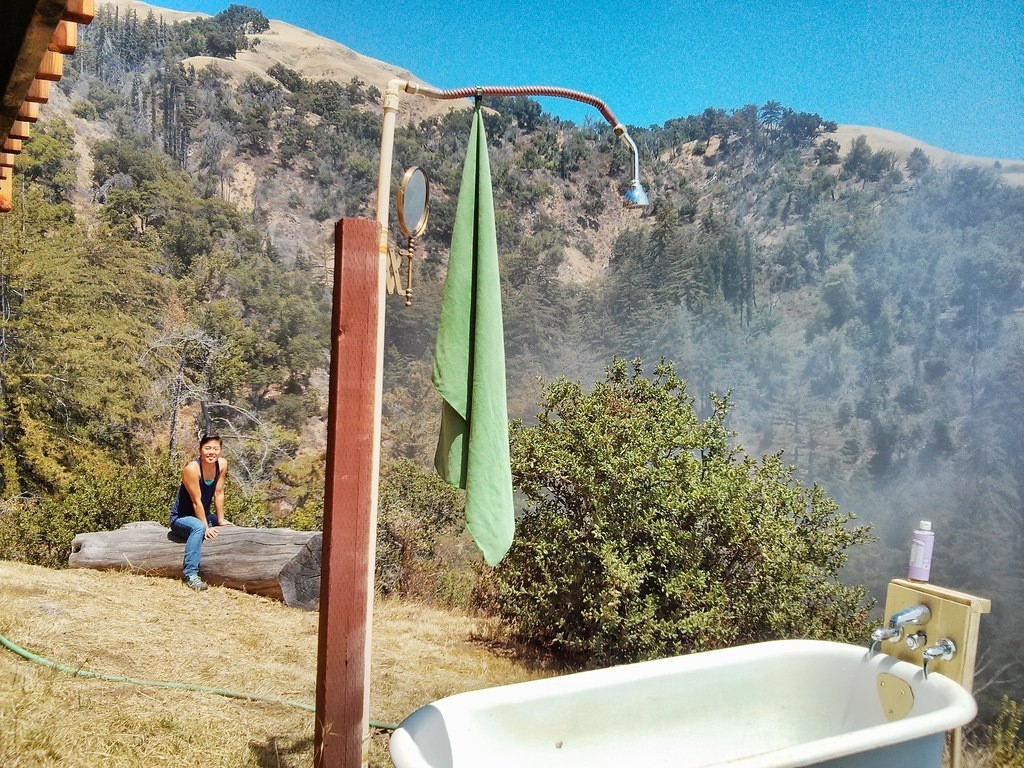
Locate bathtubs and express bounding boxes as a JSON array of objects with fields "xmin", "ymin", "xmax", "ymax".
[{"xmin": 387, "ymin": 639, "xmax": 974, "ymax": 767}]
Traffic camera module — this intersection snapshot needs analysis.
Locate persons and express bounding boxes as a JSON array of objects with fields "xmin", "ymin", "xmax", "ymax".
[{"xmin": 170, "ymin": 434, "xmax": 236, "ymax": 590}]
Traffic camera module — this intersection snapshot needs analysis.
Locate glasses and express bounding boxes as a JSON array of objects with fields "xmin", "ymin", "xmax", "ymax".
[{"xmin": 204, "ymin": 433, "xmax": 217, "ymax": 438}]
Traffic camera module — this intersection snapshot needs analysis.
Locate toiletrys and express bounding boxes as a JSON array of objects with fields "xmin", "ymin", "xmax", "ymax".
[{"xmin": 909, "ymin": 520, "xmax": 935, "ymax": 583}]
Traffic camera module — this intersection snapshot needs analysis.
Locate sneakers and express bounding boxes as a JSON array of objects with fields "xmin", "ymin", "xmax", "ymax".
[{"xmin": 183, "ymin": 573, "xmax": 208, "ymax": 591}]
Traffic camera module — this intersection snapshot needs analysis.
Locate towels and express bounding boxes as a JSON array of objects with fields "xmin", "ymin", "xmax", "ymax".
[{"xmin": 432, "ymin": 86, "xmax": 515, "ymax": 568}]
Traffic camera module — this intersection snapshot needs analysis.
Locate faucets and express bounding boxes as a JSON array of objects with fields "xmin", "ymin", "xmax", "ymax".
[{"xmin": 888, "ymin": 604, "xmax": 933, "ymax": 627}]
